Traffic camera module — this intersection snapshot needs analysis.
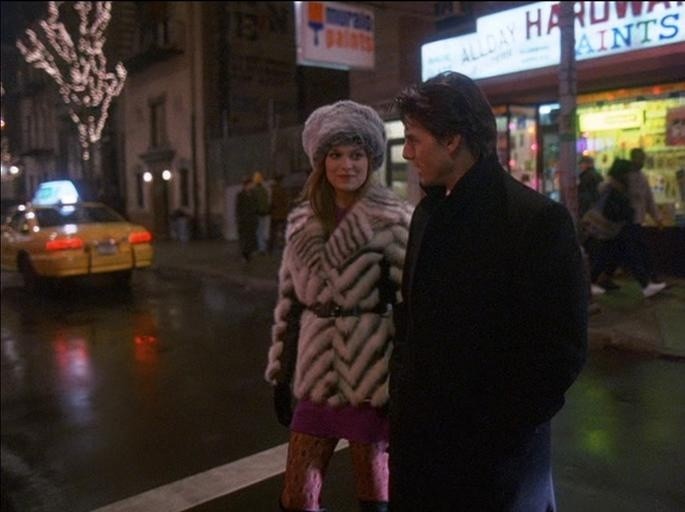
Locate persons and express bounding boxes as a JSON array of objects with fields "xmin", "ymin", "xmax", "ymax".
[
  {"xmin": 252, "ymin": 170, "xmax": 272, "ymax": 257},
  {"xmin": 235, "ymin": 177, "xmax": 258, "ymax": 265},
  {"xmin": 263, "ymin": 97, "xmax": 416, "ymax": 511},
  {"xmin": 577, "ymin": 148, "xmax": 668, "ymax": 299},
  {"xmin": 266, "ymin": 173, "xmax": 290, "ymax": 286},
  {"xmin": 388, "ymin": 72, "xmax": 590, "ymax": 511}
]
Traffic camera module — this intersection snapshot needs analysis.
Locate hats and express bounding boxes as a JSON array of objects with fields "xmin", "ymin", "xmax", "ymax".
[{"xmin": 299, "ymin": 100, "xmax": 386, "ymax": 171}]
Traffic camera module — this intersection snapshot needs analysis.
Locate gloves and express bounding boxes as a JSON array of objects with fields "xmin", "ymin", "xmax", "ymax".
[{"xmin": 270, "ymin": 373, "xmax": 298, "ymax": 429}]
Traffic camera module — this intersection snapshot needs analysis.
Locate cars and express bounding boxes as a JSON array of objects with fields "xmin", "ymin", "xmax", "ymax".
[{"xmin": 0, "ymin": 178, "xmax": 155, "ymax": 289}]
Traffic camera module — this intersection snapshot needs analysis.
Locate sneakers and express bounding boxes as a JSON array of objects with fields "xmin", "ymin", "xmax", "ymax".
[
  {"xmin": 587, "ymin": 279, "xmax": 623, "ymax": 297},
  {"xmin": 640, "ymin": 280, "xmax": 669, "ymax": 299}
]
